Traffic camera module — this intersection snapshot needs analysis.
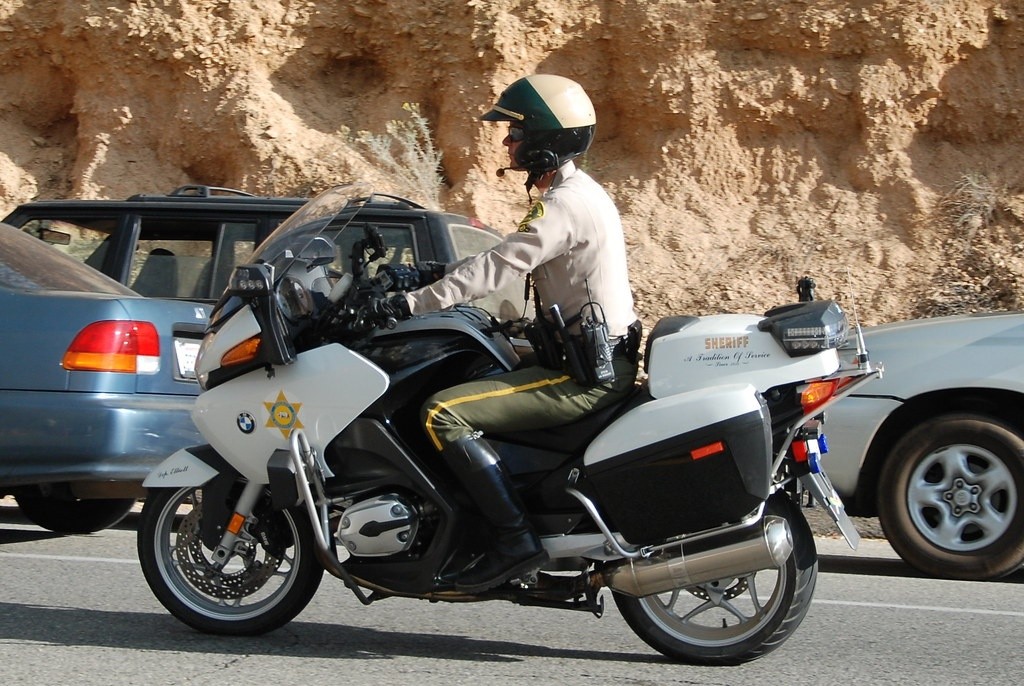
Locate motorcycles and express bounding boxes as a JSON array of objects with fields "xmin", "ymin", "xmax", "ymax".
[{"xmin": 137, "ymin": 178, "xmax": 884, "ymax": 668}]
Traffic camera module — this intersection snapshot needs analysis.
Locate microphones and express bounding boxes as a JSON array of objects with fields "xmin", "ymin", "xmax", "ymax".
[{"xmin": 497, "ymin": 166, "xmax": 524, "ymax": 178}]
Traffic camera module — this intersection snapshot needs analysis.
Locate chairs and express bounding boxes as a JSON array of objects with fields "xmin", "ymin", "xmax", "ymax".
[{"xmin": 129, "ymin": 248, "xmax": 221, "ymax": 300}]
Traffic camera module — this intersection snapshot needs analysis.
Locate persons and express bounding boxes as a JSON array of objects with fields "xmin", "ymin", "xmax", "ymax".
[{"xmin": 355, "ymin": 74, "xmax": 639, "ymax": 595}]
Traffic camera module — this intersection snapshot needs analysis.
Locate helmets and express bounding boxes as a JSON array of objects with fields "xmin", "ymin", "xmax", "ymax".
[{"xmin": 478, "ymin": 72, "xmax": 597, "ymax": 172}]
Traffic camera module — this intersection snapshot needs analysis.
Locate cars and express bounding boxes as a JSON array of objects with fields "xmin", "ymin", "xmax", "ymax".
[
  {"xmin": 819, "ymin": 314, "xmax": 1024, "ymax": 581},
  {"xmin": 0, "ymin": 225, "xmax": 227, "ymax": 536}
]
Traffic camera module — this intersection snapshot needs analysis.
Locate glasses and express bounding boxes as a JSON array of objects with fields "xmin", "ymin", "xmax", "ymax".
[{"xmin": 507, "ymin": 124, "xmax": 527, "ymax": 141}]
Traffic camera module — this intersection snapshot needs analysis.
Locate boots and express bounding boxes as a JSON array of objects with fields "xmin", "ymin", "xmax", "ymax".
[{"xmin": 442, "ymin": 430, "xmax": 551, "ymax": 594}]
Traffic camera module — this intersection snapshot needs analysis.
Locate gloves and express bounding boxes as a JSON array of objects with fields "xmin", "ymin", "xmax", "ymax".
[
  {"xmin": 377, "ymin": 263, "xmax": 446, "ymax": 290},
  {"xmin": 346, "ymin": 294, "xmax": 410, "ymax": 339}
]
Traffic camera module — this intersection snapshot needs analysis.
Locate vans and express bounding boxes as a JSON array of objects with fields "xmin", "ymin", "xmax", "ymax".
[{"xmin": 3, "ymin": 185, "xmax": 504, "ymax": 309}]
